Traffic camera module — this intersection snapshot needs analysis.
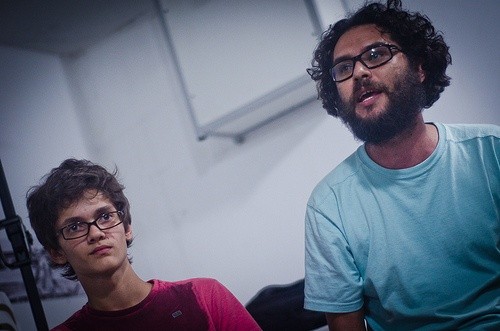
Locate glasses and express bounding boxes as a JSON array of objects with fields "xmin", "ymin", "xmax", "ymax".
[
  {"xmin": 329, "ymin": 44, "xmax": 405, "ymax": 82},
  {"xmin": 55, "ymin": 211, "xmax": 125, "ymax": 240}
]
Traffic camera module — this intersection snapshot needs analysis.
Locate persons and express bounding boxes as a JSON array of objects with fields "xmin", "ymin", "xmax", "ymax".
[
  {"xmin": 26, "ymin": 159, "xmax": 263, "ymax": 330},
  {"xmin": 301, "ymin": 0, "xmax": 500, "ymax": 331}
]
